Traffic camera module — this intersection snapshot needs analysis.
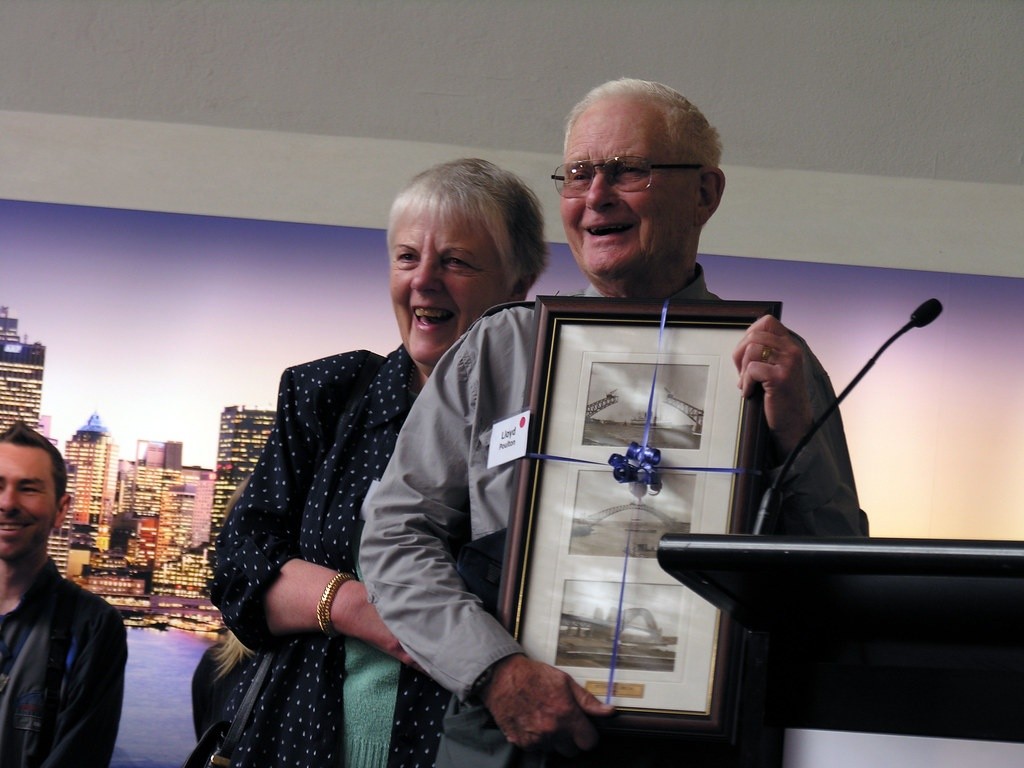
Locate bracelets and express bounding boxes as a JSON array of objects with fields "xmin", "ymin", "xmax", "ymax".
[{"xmin": 317, "ymin": 572, "xmax": 355, "ymax": 637}]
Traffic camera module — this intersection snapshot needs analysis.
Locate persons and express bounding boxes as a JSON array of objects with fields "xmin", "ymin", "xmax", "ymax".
[
  {"xmin": 216, "ymin": 153, "xmax": 545, "ymax": 768},
  {"xmin": 357, "ymin": 75, "xmax": 868, "ymax": 768},
  {"xmin": 1, "ymin": 422, "xmax": 128, "ymax": 768},
  {"xmin": 192, "ymin": 473, "xmax": 260, "ymax": 740}
]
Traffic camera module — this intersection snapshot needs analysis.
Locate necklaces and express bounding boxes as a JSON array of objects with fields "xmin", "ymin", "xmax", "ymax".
[{"xmin": 408, "ymin": 363, "xmax": 415, "ymax": 388}]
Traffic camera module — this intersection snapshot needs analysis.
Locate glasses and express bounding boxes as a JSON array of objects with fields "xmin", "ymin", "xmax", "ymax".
[{"xmin": 551, "ymin": 155, "xmax": 703, "ymax": 199}]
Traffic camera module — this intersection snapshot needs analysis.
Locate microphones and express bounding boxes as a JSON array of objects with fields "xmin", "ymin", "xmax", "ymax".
[{"xmin": 751, "ymin": 299, "xmax": 944, "ymax": 536}]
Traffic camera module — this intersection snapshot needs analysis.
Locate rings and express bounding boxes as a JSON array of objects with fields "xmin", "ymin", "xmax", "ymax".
[{"xmin": 761, "ymin": 345, "xmax": 772, "ymax": 362}]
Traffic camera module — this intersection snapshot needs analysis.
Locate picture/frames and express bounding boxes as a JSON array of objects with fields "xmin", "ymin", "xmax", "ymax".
[{"xmin": 493, "ymin": 294, "xmax": 784, "ymax": 749}]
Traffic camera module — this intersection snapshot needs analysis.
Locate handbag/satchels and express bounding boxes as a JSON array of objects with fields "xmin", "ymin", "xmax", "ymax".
[{"xmin": 181, "ymin": 721, "xmax": 241, "ymax": 768}]
